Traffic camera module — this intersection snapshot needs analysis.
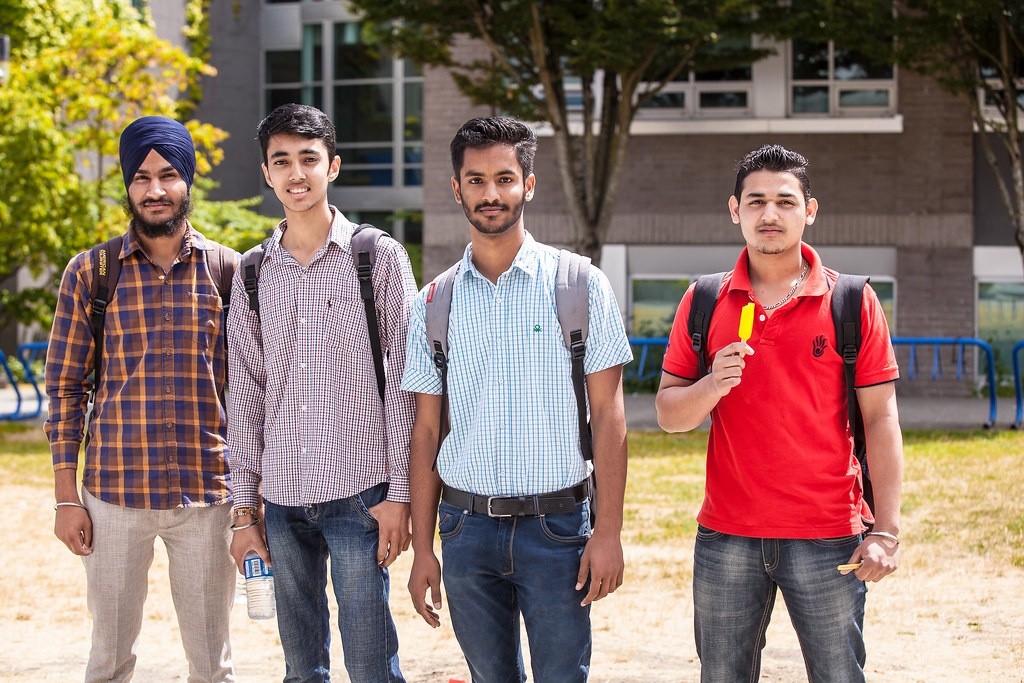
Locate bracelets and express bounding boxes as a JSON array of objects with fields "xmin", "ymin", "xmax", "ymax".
[
  {"xmin": 53, "ymin": 502, "xmax": 83, "ymax": 509},
  {"xmin": 868, "ymin": 532, "xmax": 900, "ymax": 543},
  {"xmin": 231, "ymin": 518, "xmax": 263, "ymax": 531},
  {"xmin": 234, "ymin": 509, "xmax": 255, "ymax": 516}
]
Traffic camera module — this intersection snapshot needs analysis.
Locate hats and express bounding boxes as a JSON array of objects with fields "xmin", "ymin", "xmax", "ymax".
[{"xmin": 118, "ymin": 117, "xmax": 195, "ymax": 193}]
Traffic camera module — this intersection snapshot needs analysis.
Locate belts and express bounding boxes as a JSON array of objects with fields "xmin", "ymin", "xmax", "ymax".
[{"xmin": 442, "ymin": 475, "xmax": 591, "ymax": 518}]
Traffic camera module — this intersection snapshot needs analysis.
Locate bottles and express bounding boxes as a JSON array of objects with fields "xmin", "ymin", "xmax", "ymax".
[{"xmin": 243, "ymin": 551, "xmax": 276, "ymax": 619}]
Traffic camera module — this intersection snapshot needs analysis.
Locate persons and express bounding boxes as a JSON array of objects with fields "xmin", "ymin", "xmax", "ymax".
[
  {"xmin": 43, "ymin": 116, "xmax": 241, "ymax": 683},
  {"xmin": 401, "ymin": 118, "xmax": 634, "ymax": 683},
  {"xmin": 225, "ymin": 102, "xmax": 420, "ymax": 683},
  {"xmin": 653, "ymin": 146, "xmax": 901, "ymax": 683}
]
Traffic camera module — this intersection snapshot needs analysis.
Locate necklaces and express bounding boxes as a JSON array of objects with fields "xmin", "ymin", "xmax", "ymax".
[{"xmin": 756, "ymin": 262, "xmax": 810, "ymax": 309}]
